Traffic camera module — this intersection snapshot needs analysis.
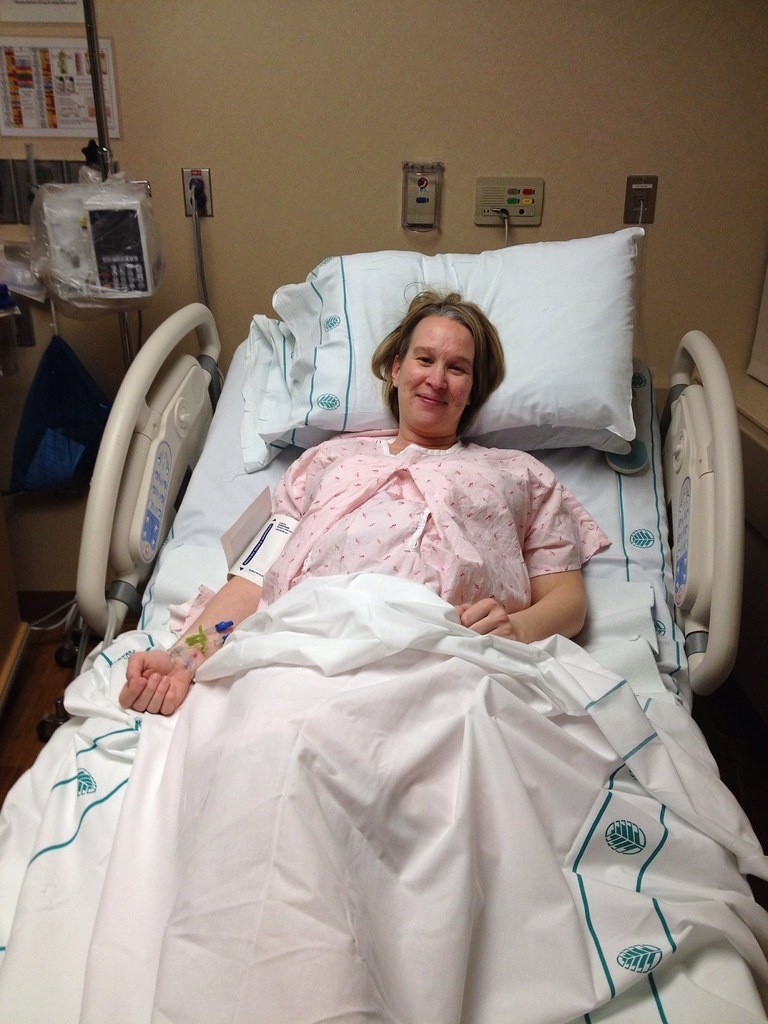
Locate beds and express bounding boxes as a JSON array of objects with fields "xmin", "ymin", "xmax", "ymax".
[{"xmin": 0, "ymin": 302, "xmax": 767, "ymax": 1023}]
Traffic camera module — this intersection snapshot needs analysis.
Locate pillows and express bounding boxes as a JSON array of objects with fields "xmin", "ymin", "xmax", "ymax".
[
  {"xmin": 241, "ymin": 314, "xmax": 631, "ymax": 473},
  {"xmin": 254, "ymin": 226, "xmax": 645, "ymax": 442}
]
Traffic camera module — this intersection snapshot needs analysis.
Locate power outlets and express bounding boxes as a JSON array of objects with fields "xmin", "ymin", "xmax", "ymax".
[
  {"xmin": 624, "ymin": 174, "xmax": 658, "ymax": 224},
  {"xmin": 182, "ymin": 168, "xmax": 213, "ymax": 217},
  {"xmin": 474, "ymin": 176, "xmax": 544, "ymax": 224}
]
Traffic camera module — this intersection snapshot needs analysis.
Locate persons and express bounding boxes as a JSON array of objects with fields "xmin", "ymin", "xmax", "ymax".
[{"xmin": 119, "ymin": 288, "xmax": 612, "ymax": 715}]
